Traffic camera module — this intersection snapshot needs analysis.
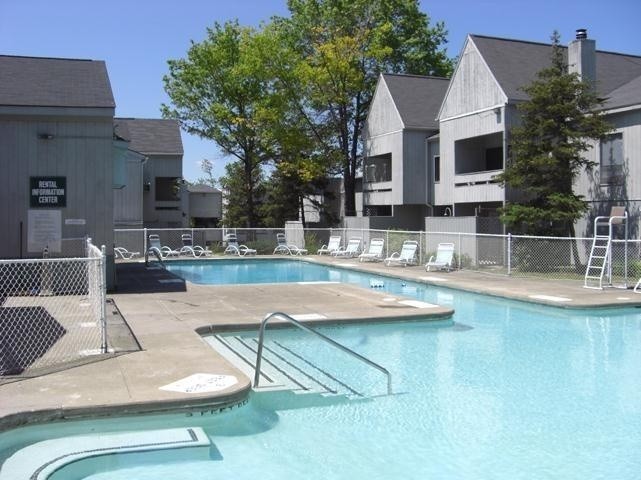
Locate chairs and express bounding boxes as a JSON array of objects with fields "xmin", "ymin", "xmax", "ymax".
[
  {"xmin": 317, "ymin": 236, "xmax": 459, "ymax": 273},
  {"xmin": 145, "ymin": 234, "xmax": 179, "ymax": 260},
  {"xmin": 179, "ymin": 233, "xmax": 212, "ymax": 258},
  {"xmin": 223, "ymin": 233, "xmax": 258, "ymax": 257},
  {"xmin": 272, "ymin": 233, "xmax": 308, "ymax": 257},
  {"xmin": 594, "ymin": 206, "xmax": 629, "ymax": 289},
  {"xmin": 113, "ymin": 247, "xmax": 141, "ymax": 261}
]
